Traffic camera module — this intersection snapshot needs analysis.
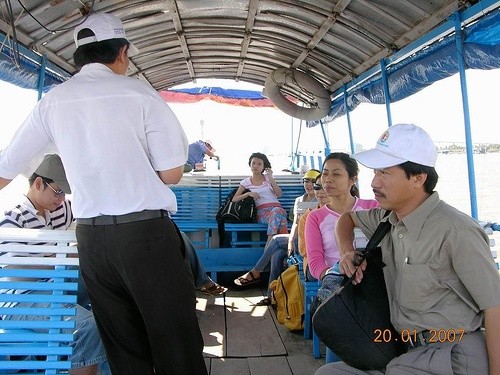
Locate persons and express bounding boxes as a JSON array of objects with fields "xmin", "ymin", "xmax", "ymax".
[
  {"xmin": 304, "ymin": 153, "xmax": 380, "ymax": 281},
  {"xmin": 0, "ymin": 14, "xmax": 209, "ymax": 375},
  {"xmin": 187, "ymin": 140, "xmax": 219, "ymax": 166},
  {"xmin": 234, "ymin": 169, "xmax": 332, "ymax": 306},
  {"xmin": 314, "ymin": 123, "xmax": 500, "ymax": 375},
  {"xmin": 232, "ymin": 153, "xmax": 288, "ymax": 247},
  {"xmin": 181, "ymin": 232, "xmax": 228, "ymax": 303},
  {"xmin": 0, "ymin": 153, "xmax": 111, "ymax": 375}
]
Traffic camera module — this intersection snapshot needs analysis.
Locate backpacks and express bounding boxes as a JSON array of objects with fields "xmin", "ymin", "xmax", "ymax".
[
  {"xmin": 270, "ymin": 263, "xmax": 305, "ymax": 331},
  {"xmin": 216, "ymin": 188, "xmax": 256, "ymax": 222},
  {"xmin": 310, "ymin": 210, "xmax": 408, "ymax": 370}
]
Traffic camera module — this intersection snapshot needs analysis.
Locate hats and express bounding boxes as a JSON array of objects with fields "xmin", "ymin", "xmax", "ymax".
[
  {"xmin": 352, "ymin": 124, "xmax": 438, "ymax": 169},
  {"xmin": 34, "ymin": 154, "xmax": 72, "ymax": 194},
  {"xmin": 303, "ymin": 170, "xmax": 320, "ymax": 180},
  {"xmin": 73, "ymin": 12, "xmax": 140, "ymax": 57}
]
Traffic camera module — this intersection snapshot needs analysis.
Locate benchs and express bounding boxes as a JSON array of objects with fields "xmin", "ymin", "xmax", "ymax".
[{"xmin": 0, "ymin": 186, "xmax": 347, "ymax": 375}]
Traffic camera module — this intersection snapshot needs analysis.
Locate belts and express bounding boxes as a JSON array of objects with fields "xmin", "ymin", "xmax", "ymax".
[
  {"xmin": 404, "ymin": 326, "xmax": 482, "ymax": 351},
  {"xmin": 77, "ymin": 210, "xmax": 168, "ymax": 225}
]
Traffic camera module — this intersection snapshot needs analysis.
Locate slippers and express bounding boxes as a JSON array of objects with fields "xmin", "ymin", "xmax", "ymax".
[
  {"xmin": 234, "ymin": 271, "xmax": 261, "ymax": 286},
  {"xmin": 257, "ymin": 298, "xmax": 272, "ymax": 306},
  {"xmin": 201, "ymin": 283, "xmax": 228, "ymax": 296}
]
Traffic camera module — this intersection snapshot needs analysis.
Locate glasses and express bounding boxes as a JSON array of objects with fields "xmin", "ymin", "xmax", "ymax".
[
  {"xmin": 43, "ymin": 180, "xmax": 63, "ymax": 197},
  {"xmin": 313, "ymin": 184, "xmax": 322, "ymax": 190},
  {"xmin": 303, "ymin": 179, "xmax": 316, "ymax": 183}
]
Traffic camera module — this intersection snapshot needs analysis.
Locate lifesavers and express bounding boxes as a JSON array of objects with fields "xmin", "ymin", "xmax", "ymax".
[{"xmin": 264, "ymin": 67, "xmax": 331, "ymax": 121}]
